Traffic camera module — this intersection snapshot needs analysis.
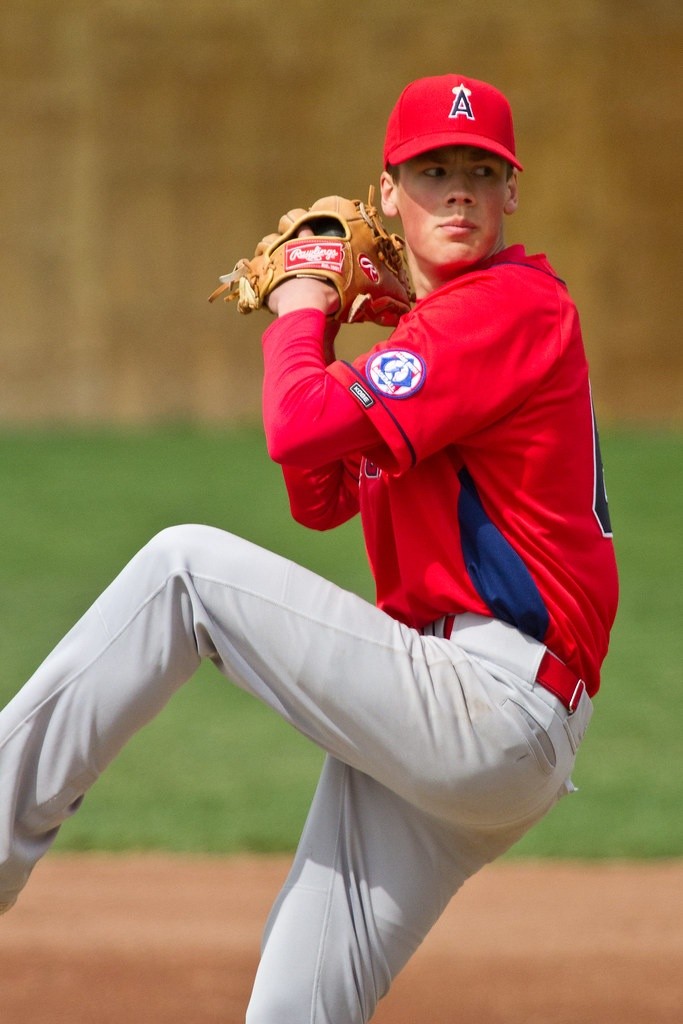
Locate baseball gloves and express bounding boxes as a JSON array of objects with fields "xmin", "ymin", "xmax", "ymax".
[{"xmin": 206, "ymin": 181, "xmax": 419, "ymax": 331}]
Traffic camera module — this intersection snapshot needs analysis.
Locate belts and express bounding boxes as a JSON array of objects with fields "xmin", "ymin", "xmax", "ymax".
[{"xmin": 422, "ymin": 615, "xmax": 586, "ymax": 715}]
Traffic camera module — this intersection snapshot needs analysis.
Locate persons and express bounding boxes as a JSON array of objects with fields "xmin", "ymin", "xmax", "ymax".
[{"xmin": 1, "ymin": 73, "xmax": 620, "ymax": 1024}]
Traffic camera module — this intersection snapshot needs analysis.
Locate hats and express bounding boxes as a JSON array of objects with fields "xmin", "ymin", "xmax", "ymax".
[{"xmin": 383, "ymin": 72, "xmax": 524, "ymax": 173}]
